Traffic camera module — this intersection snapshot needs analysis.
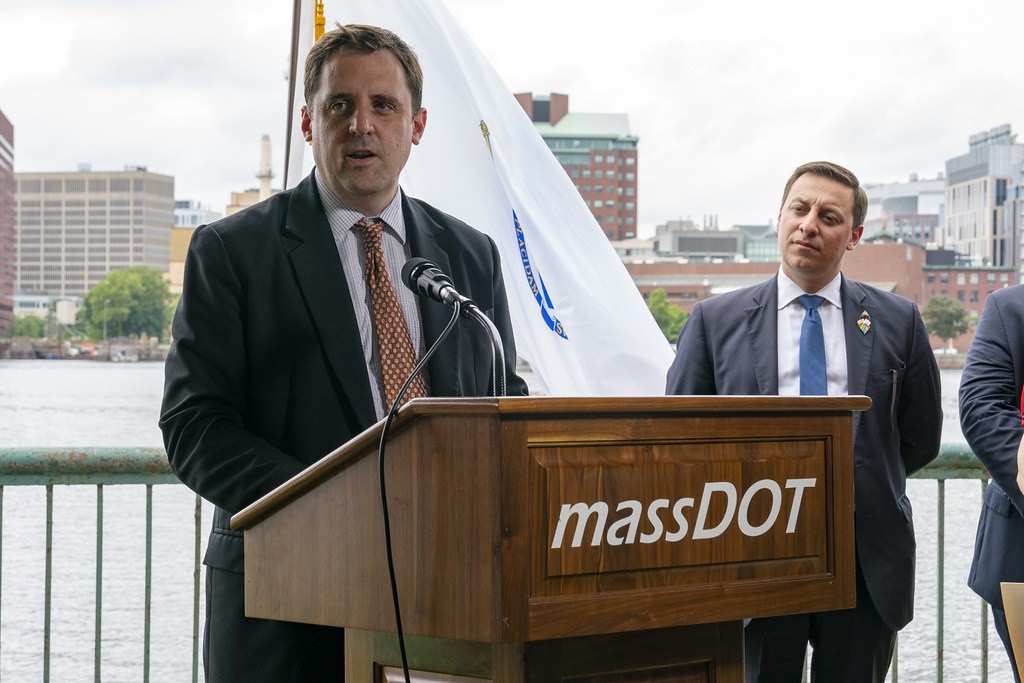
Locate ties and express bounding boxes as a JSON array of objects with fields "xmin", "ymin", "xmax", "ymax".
[
  {"xmin": 352, "ymin": 219, "xmax": 429, "ymax": 413},
  {"xmin": 797, "ymin": 294, "xmax": 828, "ymax": 397}
]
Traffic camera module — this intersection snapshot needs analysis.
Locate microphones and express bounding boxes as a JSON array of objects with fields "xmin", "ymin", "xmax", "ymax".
[{"xmin": 401, "ymin": 257, "xmax": 461, "ymax": 304}]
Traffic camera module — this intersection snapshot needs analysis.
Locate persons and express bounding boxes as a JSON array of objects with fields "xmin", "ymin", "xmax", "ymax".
[
  {"xmin": 158, "ymin": 21, "xmax": 531, "ymax": 683},
  {"xmin": 958, "ymin": 282, "xmax": 1024, "ymax": 683},
  {"xmin": 663, "ymin": 161, "xmax": 942, "ymax": 683}
]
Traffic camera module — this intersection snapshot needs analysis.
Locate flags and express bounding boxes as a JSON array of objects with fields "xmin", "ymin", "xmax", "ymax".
[{"xmin": 307, "ymin": 0, "xmax": 682, "ymax": 398}]
